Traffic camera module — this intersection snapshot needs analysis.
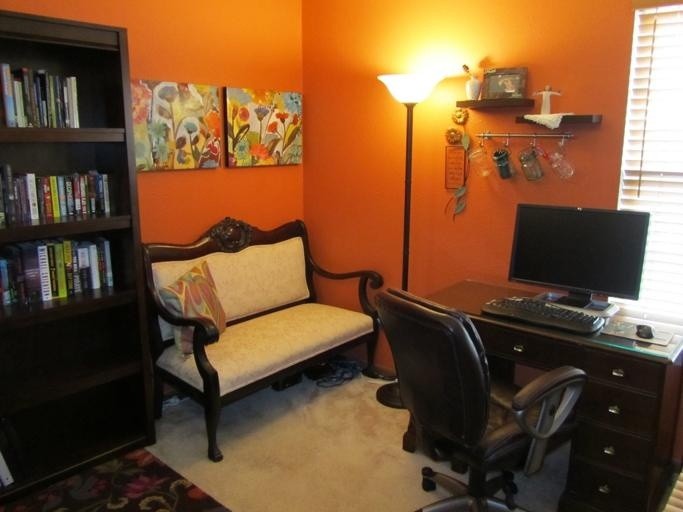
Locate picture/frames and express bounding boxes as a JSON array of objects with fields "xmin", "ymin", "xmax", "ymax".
[
  {"xmin": 481, "ymin": 66, "xmax": 528, "ymax": 99},
  {"xmin": 445, "ymin": 145, "xmax": 466, "ymax": 189}
]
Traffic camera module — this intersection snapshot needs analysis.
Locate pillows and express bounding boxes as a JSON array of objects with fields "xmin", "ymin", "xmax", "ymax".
[{"xmin": 151, "ymin": 259, "xmax": 226, "ymax": 358}]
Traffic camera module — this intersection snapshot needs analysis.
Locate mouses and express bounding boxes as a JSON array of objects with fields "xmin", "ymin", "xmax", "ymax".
[{"xmin": 636, "ymin": 324, "xmax": 653, "ymax": 340}]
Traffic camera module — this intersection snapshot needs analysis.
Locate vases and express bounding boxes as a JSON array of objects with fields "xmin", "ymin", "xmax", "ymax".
[{"xmin": 466, "ymin": 76, "xmax": 481, "ymax": 101}]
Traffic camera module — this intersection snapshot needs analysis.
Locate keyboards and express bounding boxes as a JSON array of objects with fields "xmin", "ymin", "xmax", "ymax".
[{"xmin": 482, "ymin": 296, "xmax": 606, "ymax": 334}]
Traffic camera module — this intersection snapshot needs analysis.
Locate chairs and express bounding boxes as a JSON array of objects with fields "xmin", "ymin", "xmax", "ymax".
[{"xmin": 382, "ymin": 288, "xmax": 588, "ymax": 512}]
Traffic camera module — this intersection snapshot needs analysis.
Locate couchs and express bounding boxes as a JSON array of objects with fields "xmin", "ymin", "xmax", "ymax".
[{"xmin": 142, "ymin": 217, "xmax": 381, "ymax": 463}]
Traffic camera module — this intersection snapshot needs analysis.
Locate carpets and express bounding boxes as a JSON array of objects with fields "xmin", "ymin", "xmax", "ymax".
[
  {"xmin": 145, "ymin": 361, "xmax": 572, "ymax": 512},
  {"xmin": 0, "ymin": 447, "xmax": 230, "ymax": 512}
]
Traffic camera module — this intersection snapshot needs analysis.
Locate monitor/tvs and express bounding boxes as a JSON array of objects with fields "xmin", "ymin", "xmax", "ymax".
[{"xmin": 507, "ymin": 204, "xmax": 650, "ymax": 310}]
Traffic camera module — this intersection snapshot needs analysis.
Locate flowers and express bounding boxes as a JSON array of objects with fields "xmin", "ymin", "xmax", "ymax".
[{"xmin": 443, "ymin": 107, "xmax": 471, "ymax": 226}]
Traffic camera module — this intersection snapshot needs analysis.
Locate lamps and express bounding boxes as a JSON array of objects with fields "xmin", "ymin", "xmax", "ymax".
[{"xmin": 378, "ymin": 74, "xmax": 442, "ymax": 408}]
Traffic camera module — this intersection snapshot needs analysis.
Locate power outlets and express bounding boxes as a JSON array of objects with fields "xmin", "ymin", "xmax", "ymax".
[{"xmin": 337, "ymin": 360, "xmax": 364, "ymax": 371}]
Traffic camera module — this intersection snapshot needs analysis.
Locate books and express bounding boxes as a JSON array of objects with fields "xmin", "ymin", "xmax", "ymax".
[
  {"xmin": 2, "ymin": 236, "xmax": 114, "ymax": 307},
  {"xmin": 2, "ymin": 63, "xmax": 79, "ymax": 130},
  {"xmin": 1, "ymin": 453, "xmax": 14, "ymax": 488},
  {"xmin": 1, "ymin": 162, "xmax": 109, "ymax": 222}
]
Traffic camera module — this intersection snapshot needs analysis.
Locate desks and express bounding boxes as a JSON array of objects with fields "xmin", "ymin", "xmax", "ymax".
[{"xmin": 403, "ymin": 280, "xmax": 683, "ymax": 512}]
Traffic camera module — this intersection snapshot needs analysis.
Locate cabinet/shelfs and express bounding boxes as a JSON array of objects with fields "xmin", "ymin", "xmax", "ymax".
[{"xmin": 0, "ymin": 9, "xmax": 157, "ymax": 494}]
